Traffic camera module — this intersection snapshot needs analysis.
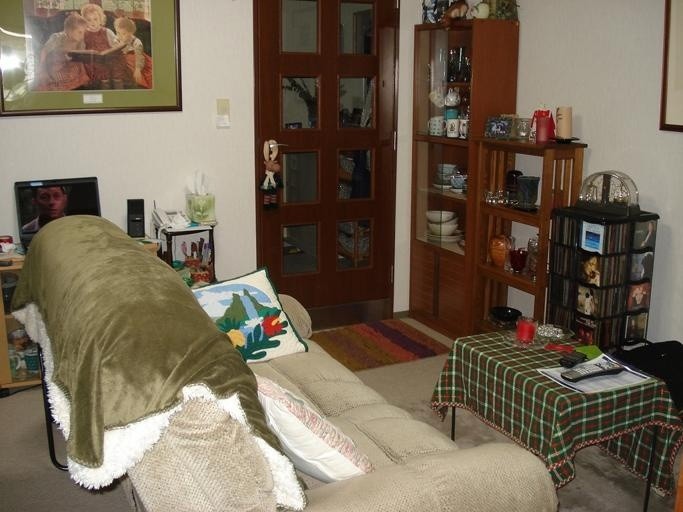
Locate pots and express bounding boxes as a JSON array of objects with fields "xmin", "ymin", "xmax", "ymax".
[{"xmin": 489, "ymin": 303, "xmax": 522, "ymax": 327}]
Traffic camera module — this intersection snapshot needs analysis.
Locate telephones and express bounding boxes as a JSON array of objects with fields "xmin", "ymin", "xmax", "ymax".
[{"xmin": 152, "ymin": 208, "xmax": 191, "ymax": 230}]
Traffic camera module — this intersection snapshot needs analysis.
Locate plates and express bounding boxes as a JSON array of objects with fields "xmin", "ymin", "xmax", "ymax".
[
  {"xmin": 548, "ymin": 137, "xmax": 580, "ymax": 144},
  {"xmin": 538, "ymin": 324, "xmax": 574, "ymax": 344}
]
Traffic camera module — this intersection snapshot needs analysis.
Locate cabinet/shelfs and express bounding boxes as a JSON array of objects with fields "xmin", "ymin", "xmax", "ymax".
[
  {"xmin": 409, "ymin": 19, "xmax": 520, "ymax": 340},
  {"xmin": 550, "ymin": 206, "xmax": 659, "ymax": 356},
  {"xmin": 475, "ymin": 137, "xmax": 588, "ymax": 337},
  {"xmin": 0, "ymin": 239, "xmax": 157, "ymax": 396}
]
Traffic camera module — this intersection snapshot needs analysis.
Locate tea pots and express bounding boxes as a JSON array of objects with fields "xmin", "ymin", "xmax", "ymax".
[
  {"xmin": 428, "ymin": 116, "xmax": 447, "ymax": 136},
  {"xmin": 484, "ymin": 190, "xmax": 504, "ymax": 209}
]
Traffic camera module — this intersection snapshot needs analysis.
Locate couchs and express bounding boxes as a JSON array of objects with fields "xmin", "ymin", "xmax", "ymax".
[{"xmin": 9, "ymin": 214, "xmax": 561, "ymax": 512}]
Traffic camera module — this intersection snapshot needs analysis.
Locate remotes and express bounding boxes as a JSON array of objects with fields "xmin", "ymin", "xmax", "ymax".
[
  {"xmin": 559, "ymin": 352, "xmax": 587, "ymax": 368},
  {"xmin": 560, "ymin": 363, "xmax": 623, "ymax": 382}
]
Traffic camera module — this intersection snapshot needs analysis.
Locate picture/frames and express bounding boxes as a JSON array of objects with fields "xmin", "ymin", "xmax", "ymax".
[
  {"xmin": 0, "ymin": 0, "xmax": 183, "ymax": 115},
  {"xmin": 659, "ymin": 0, "xmax": 682, "ymax": 133}
]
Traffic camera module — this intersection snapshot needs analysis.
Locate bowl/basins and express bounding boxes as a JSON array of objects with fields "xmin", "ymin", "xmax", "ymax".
[
  {"xmin": 507, "ymin": 170, "xmax": 523, "ymax": 199},
  {"xmin": 432, "ymin": 163, "xmax": 468, "ymax": 188},
  {"xmin": 442, "ymin": 109, "xmax": 460, "ymax": 119},
  {"xmin": 425, "ymin": 209, "xmax": 465, "ymax": 250}
]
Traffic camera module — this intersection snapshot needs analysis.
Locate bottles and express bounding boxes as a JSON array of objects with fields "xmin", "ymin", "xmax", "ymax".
[{"xmin": 531, "ymin": 110, "xmax": 556, "ymax": 143}]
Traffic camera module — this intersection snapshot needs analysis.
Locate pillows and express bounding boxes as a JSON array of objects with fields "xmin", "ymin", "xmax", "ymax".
[
  {"xmin": 194, "ymin": 265, "xmax": 308, "ymax": 365},
  {"xmin": 254, "ymin": 376, "xmax": 375, "ymax": 484}
]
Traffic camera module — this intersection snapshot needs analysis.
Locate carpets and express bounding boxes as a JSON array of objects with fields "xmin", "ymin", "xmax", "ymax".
[{"xmin": 309, "ymin": 318, "xmax": 451, "ymax": 372}]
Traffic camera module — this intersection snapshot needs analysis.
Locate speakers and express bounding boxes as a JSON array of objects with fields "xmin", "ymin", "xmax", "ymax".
[{"xmin": 126, "ymin": 198, "xmax": 145, "ymax": 238}]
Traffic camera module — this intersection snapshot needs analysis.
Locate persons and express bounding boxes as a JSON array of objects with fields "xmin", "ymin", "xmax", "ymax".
[
  {"xmin": 259, "ymin": 140, "xmax": 283, "ymax": 210},
  {"xmin": 22, "ymin": 187, "xmax": 68, "ymax": 237},
  {"xmin": 40, "ymin": 4, "xmax": 152, "ymax": 89}
]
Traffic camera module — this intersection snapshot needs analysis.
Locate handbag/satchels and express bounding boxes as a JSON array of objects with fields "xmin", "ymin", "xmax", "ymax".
[{"xmin": 611, "ymin": 337, "xmax": 682, "ymax": 412}]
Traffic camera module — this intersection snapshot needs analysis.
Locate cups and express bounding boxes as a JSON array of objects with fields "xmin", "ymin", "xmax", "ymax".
[
  {"xmin": 0, "ymin": 235, "xmax": 12, "ymax": 253},
  {"xmin": 446, "ymin": 119, "xmax": 459, "ymax": 138},
  {"xmin": 7, "ymin": 348, "xmax": 24, "ymax": 378},
  {"xmin": 444, "ymin": 88, "xmax": 461, "ymax": 107},
  {"xmin": 517, "ymin": 318, "xmax": 538, "ymax": 345},
  {"xmin": 429, "ymin": 88, "xmax": 444, "ymax": 108},
  {"xmin": 478, "ymin": 113, "xmax": 531, "ymax": 144},
  {"xmin": 459, "ymin": 119, "xmax": 469, "ymax": 140},
  {"xmin": 556, "ymin": 107, "xmax": 572, "ymax": 137},
  {"xmin": 510, "ymin": 248, "xmax": 528, "ymax": 273},
  {"xmin": 24, "ymin": 351, "xmax": 40, "ymax": 373}
]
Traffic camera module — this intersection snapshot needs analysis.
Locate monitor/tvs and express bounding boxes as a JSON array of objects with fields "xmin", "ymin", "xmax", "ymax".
[{"xmin": 14, "ymin": 176, "xmax": 100, "ymax": 248}]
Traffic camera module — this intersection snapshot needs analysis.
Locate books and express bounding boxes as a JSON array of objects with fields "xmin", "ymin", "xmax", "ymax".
[{"xmin": 550, "ymin": 215, "xmax": 656, "ymax": 348}]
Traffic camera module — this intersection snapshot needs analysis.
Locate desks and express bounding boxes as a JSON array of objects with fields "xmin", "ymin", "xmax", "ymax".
[{"xmin": 430, "ymin": 326, "xmax": 659, "ymax": 512}]
